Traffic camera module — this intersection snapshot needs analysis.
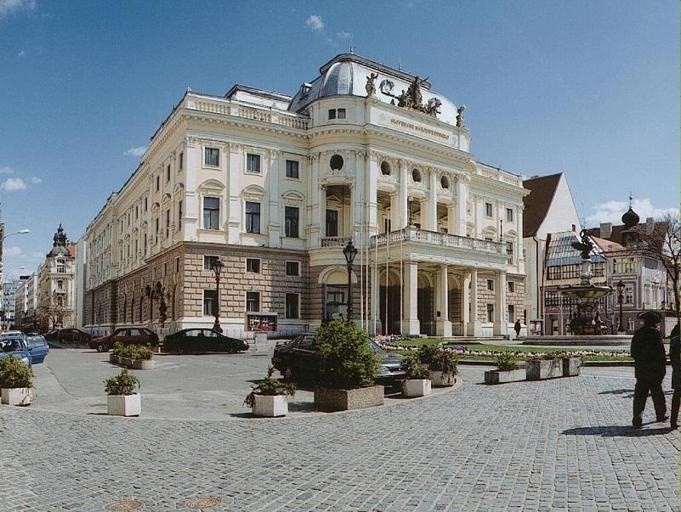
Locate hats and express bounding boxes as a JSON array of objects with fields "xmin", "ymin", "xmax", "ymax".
[{"xmin": 636, "ymin": 310, "xmax": 664, "ymax": 323}]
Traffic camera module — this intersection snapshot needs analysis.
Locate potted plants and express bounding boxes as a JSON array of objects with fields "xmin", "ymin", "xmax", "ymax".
[
  {"xmin": 399, "ymin": 357, "xmax": 432, "ymax": 396},
  {"xmin": 243, "ymin": 364, "xmax": 296, "ymax": 416},
  {"xmin": 429, "ymin": 347, "xmax": 460, "ymax": 386},
  {"xmin": 1, "ymin": 354, "xmax": 34, "ymax": 405},
  {"xmin": 133, "ymin": 348, "xmax": 154, "ymax": 370},
  {"xmin": 110, "ymin": 341, "xmax": 123, "ymax": 362},
  {"xmin": 121, "ymin": 344, "xmax": 140, "ymax": 367},
  {"xmin": 528, "ymin": 349, "xmax": 564, "ymax": 379},
  {"xmin": 485, "ymin": 349, "xmax": 526, "ymax": 383},
  {"xmin": 566, "ymin": 348, "xmax": 582, "ymax": 377},
  {"xmin": 103, "ymin": 370, "xmax": 142, "ymax": 417}
]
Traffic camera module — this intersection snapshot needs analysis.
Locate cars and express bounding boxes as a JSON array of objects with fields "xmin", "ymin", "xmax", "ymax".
[
  {"xmin": 0, "ymin": 336, "xmax": 49, "ymax": 369},
  {"xmin": 272, "ymin": 334, "xmax": 406, "ymax": 391},
  {"xmin": 88, "ymin": 328, "xmax": 159, "ymax": 352},
  {"xmin": 58, "ymin": 328, "xmax": 90, "ymax": 344},
  {"xmin": 164, "ymin": 328, "xmax": 249, "ymax": 354}
]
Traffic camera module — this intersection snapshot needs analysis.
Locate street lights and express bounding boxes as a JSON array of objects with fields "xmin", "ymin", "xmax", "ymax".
[
  {"xmin": 0, "ymin": 229, "xmax": 30, "ymax": 242},
  {"xmin": 343, "ymin": 238, "xmax": 358, "ymax": 322},
  {"xmin": 4, "ymin": 267, "xmax": 24, "ymax": 330},
  {"xmin": 211, "ymin": 257, "xmax": 225, "ymax": 333},
  {"xmin": 616, "ymin": 280, "xmax": 625, "ymax": 331}
]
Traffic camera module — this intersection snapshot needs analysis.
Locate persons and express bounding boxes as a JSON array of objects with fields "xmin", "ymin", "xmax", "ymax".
[
  {"xmin": 668, "ymin": 311, "xmax": 681, "ymax": 430},
  {"xmin": 365, "ymin": 71, "xmax": 380, "ymax": 99},
  {"xmin": 395, "ymin": 74, "xmax": 442, "ymax": 119},
  {"xmin": 630, "ymin": 310, "xmax": 669, "ymax": 429}
]
